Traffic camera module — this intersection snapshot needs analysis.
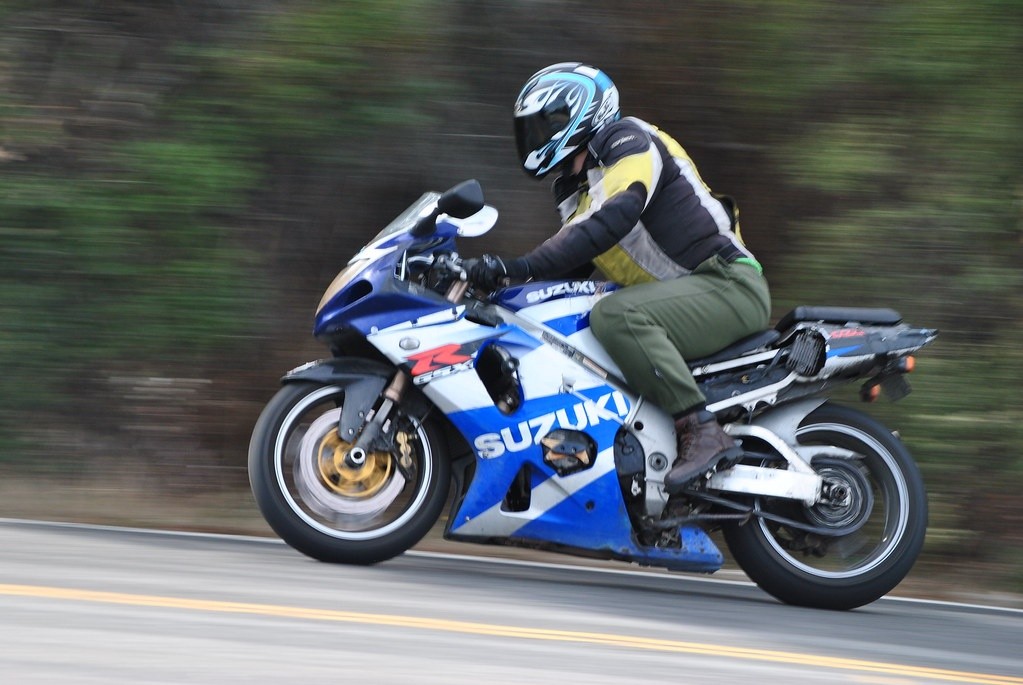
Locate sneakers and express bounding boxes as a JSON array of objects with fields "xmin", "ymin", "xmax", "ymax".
[{"xmin": 665, "ymin": 411, "xmax": 747, "ymax": 493}]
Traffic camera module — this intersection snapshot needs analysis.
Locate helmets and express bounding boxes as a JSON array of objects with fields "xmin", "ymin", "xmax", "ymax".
[{"xmin": 514, "ymin": 63, "xmax": 624, "ymax": 181}]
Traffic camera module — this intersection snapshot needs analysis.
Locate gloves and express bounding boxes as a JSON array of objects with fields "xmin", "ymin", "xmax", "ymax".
[{"xmin": 468, "ymin": 254, "xmax": 532, "ymax": 293}]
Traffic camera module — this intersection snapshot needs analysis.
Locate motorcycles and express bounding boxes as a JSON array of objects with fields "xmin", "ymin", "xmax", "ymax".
[{"xmin": 247, "ymin": 176, "xmax": 941, "ymax": 612}]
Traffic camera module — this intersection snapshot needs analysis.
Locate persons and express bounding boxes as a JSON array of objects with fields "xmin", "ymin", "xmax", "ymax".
[{"xmin": 463, "ymin": 61, "xmax": 772, "ymax": 490}]
